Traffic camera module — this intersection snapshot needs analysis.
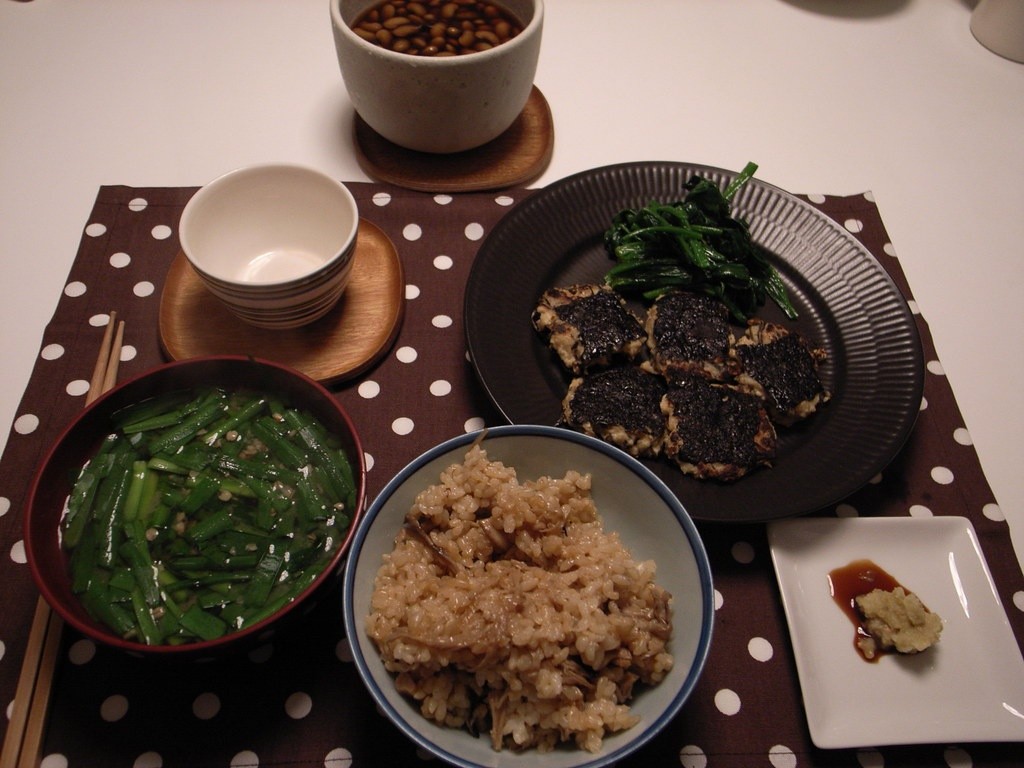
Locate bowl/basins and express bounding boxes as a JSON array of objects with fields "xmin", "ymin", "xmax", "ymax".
[
  {"xmin": 343, "ymin": 425, "xmax": 715, "ymax": 768},
  {"xmin": 24, "ymin": 355, "xmax": 367, "ymax": 658},
  {"xmin": 178, "ymin": 162, "xmax": 358, "ymax": 328},
  {"xmin": 329, "ymin": 0, "xmax": 544, "ymax": 154}
]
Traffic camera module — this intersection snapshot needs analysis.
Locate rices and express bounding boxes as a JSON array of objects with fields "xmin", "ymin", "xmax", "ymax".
[{"xmin": 365, "ymin": 444, "xmax": 677, "ymax": 756}]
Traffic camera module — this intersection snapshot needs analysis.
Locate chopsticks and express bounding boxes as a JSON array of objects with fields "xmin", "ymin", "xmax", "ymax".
[{"xmin": 0, "ymin": 311, "xmax": 126, "ymax": 768}]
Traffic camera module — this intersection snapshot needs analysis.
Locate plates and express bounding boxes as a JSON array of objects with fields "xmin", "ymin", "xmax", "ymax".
[
  {"xmin": 766, "ymin": 515, "xmax": 1024, "ymax": 748},
  {"xmin": 463, "ymin": 161, "xmax": 928, "ymax": 523}
]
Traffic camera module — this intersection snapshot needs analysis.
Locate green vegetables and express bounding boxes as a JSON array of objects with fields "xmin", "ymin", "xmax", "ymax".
[
  {"xmin": 603, "ymin": 159, "xmax": 799, "ymax": 326},
  {"xmin": 60, "ymin": 395, "xmax": 357, "ymax": 647}
]
